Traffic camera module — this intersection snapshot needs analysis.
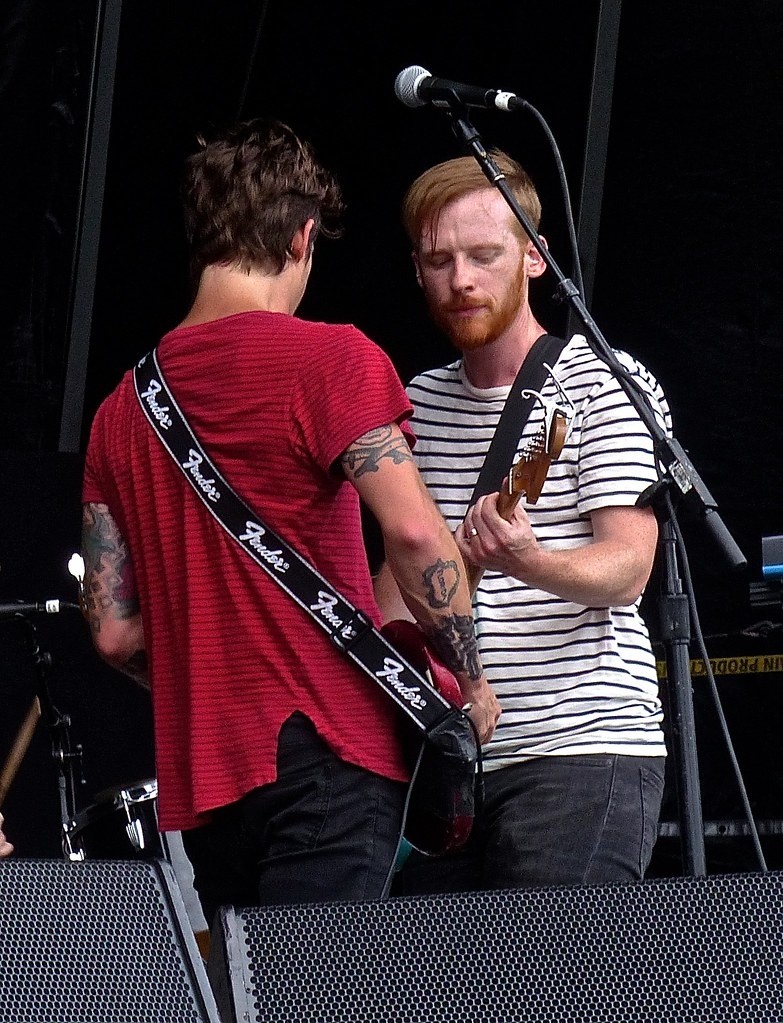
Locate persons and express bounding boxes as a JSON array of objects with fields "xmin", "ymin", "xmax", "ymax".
[
  {"xmin": 372, "ymin": 147, "xmax": 672, "ymax": 890},
  {"xmin": 83, "ymin": 118, "xmax": 502, "ymax": 1023},
  {"xmin": 0, "ymin": 812, "xmax": 14, "ymax": 856}
]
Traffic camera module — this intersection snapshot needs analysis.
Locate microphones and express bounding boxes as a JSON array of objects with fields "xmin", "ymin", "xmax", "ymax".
[{"xmin": 395, "ymin": 65, "xmax": 526, "ymax": 111}]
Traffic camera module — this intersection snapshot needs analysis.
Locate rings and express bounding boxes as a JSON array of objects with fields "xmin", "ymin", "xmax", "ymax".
[{"xmin": 466, "ymin": 528, "xmax": 478, "ymax": 539}]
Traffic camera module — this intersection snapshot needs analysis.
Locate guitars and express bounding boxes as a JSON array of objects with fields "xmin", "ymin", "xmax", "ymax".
[
  {"xmin": 380, "ymin": 411, "xmax": 568, "ymax": 768},
  {"xmin": 66, "ymin": 551, "xmax": 477, "ymax": 859}
]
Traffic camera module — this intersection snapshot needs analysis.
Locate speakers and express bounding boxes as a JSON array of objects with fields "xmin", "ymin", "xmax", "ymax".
[
  {"xmin": 0, "ymin": 856, "xmax": 222, "ymax": 1023},
  {"xmin": 210, "ymin": 869, "xmax": 783, "ymax": 1023}
]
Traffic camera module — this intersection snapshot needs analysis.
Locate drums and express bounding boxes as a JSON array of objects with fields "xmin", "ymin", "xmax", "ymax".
[{"xmin": 58, "ymin": 776, "xmax": 173, "ymax": 866}]
